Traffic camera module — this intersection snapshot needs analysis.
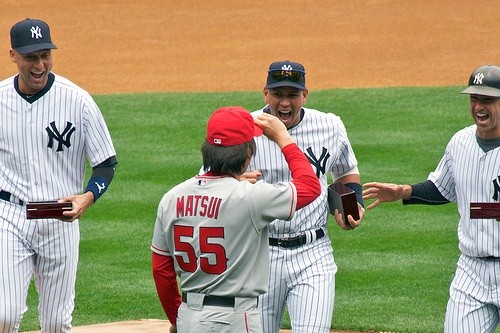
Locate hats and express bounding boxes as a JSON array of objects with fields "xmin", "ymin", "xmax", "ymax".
[
  {"xmin": 204, "ymin": 106, "xmax": 263, "ymax": 146},
  {"xmin": 460, "ymin": 65, "xmax": 500, "ymax": 97},
  {"xmin": 10, "ymin": 17, "xmax": 57, "ymax": 54},
  {"xmin": 266, "ymin": 60, "xmax": 307, "ymax": 91}
]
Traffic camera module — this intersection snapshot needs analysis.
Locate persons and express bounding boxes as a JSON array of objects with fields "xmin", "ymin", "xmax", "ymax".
[
  {"xmin": 362, "ymin": 65, "xmax": 500, "ymax": 333},
  {"xmin": 150, "ymin": 106, "xmax": 322, "ymax": 333},
  {"xmin": 198, "ymin": 58, "xmax": 366, "ymax": 333},
  {"xmin": 0, "ymin": 18, "xmax": 119, "ymax": 333}
]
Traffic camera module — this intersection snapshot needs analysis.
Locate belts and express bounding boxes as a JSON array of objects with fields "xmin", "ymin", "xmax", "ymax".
[
  {"xmin": 182, "ymin": 290, "xmax": 235, "ymax": 308},
  {"xmin": 0, "ymin": 190, "xmax": 23, "ymax": 206},
  {"xmin": 269, "ymin": 228, "xmax": 324, "ymax": 250}
]
